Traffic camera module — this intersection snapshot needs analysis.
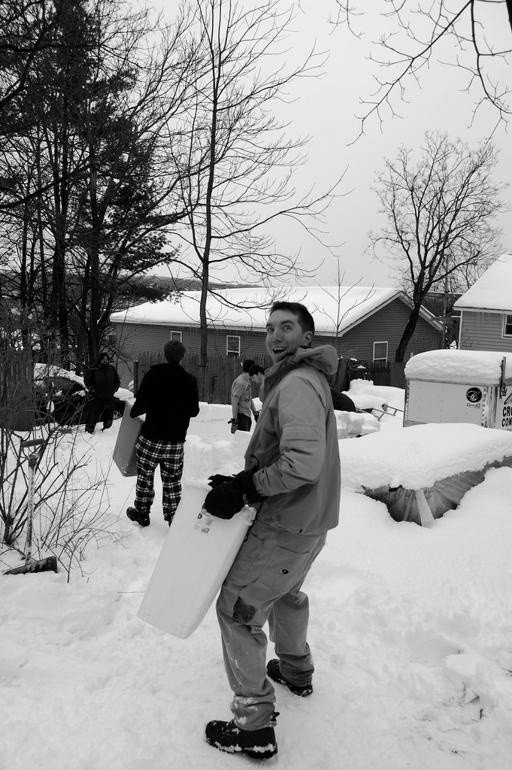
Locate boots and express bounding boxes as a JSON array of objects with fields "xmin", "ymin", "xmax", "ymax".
[{"xmin": 126, "ymin": 500, "xmax": 153, "ymax": 526}]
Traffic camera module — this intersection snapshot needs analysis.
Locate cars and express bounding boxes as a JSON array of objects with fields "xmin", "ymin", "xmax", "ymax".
[{"xmin": 34, "ymin": 362, "xmax": 135, "ymax": 426}]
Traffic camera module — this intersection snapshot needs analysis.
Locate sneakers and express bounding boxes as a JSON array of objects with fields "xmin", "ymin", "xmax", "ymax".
[
  {"xmin": 206, "ymin": 712, "xmax": 280, "ymax": 760},
  {"xmin": 266, "ymin": 659, "xmax": 313, "ymax": 697}
]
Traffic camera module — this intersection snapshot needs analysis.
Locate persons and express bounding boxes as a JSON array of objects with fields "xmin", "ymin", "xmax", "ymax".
[
  {"xmin": 202, "ymin": 300, "xmax": 342, "ymax": 760},
  {"xmin": 124, "ymin": 338, "xmax": 201, "ymax": 529},
  {"xmin": 82, "ymin": 350, "xmax": 122, "ymax": 434},
  {"xmin": 227, "ymin": 361, "xmax": 261, "ymax": 434},
  {"xmin": 249, "ymin": 363, "xmax": 268, "ymax": 402}
]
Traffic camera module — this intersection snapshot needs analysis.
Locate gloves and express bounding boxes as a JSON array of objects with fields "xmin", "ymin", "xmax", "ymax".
[{"xmin": 202, "ymin": 470, "xmax": 259, "ymax": 519}]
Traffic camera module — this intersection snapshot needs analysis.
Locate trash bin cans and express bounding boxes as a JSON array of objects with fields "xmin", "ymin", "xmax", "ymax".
[
  {"xmin": 137, "ymin": 482, "xmax": 256, "ymax": 639},
  {"xmin": 113, "ymin": 400, "xmax": 145, "ymax": 476}
]
